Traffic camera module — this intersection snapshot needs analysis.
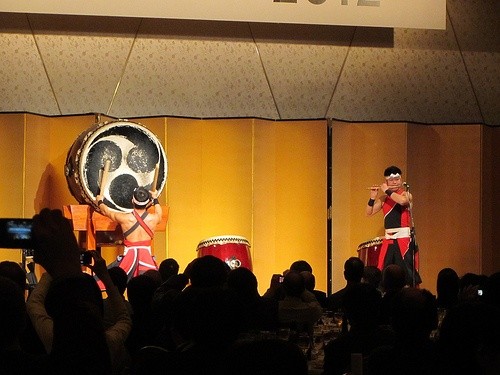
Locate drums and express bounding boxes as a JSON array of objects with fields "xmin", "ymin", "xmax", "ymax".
[
  {"xmin": 196, "ymin": 235, "xmax": 253, "ymax": 272},
  {"xmin": 64, "ymin": 118, "xmax": 168, "ymax": 215},
  {"xmin": 356, "ymin": 236, "xmax": 386, "ymax": 267}
]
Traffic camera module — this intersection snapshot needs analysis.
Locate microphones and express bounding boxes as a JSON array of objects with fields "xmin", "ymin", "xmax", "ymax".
[{"xmin": 402, "ymin": 182, "xmax": 410, "ymax": 188}]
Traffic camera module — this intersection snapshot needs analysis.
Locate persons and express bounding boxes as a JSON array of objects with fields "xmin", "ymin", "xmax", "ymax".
[
  {"xmin": 366, "ymin": 166, "xmax": 422, "ymax": 288},
  {"xmin": 0, "ymin": 254, "xmax": 500, "ymax": 375},
  {"xmin": 92, "ymin": 187, "xmax": 162, "ymax": 290}
]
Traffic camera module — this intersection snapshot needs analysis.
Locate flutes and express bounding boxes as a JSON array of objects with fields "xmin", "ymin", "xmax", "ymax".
[{"xmin": 366, "ymin": 184, "xmax": 401, "ymax": 189}]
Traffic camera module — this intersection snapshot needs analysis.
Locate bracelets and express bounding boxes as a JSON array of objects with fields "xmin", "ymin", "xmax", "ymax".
[
  {"xmin": 98, "ymin": 200, "xmax": 102, "ymax": 206},
  {"xmin": 154, "ymin": 199, "xmax": 159, "ymax": 205},
  {"xmin": 385, "ymin": 188, "xmax": 393, "ymax": 196},
  {"xmin": 368, "ymin": 198, "xmax": 375, "ymax": 206}
]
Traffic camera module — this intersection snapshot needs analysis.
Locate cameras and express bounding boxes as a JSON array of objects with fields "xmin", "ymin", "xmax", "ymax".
[
  {"xmin": 279, "ymin": 276, "xmax": 285, "ymax": 286},
  {"xmin": 79, "ymin": 251, "xmax": 92, "ymax": 264},
  {"xmin": 0, "ymin": 218, "xmax": 35, "ymax": 249}
]
